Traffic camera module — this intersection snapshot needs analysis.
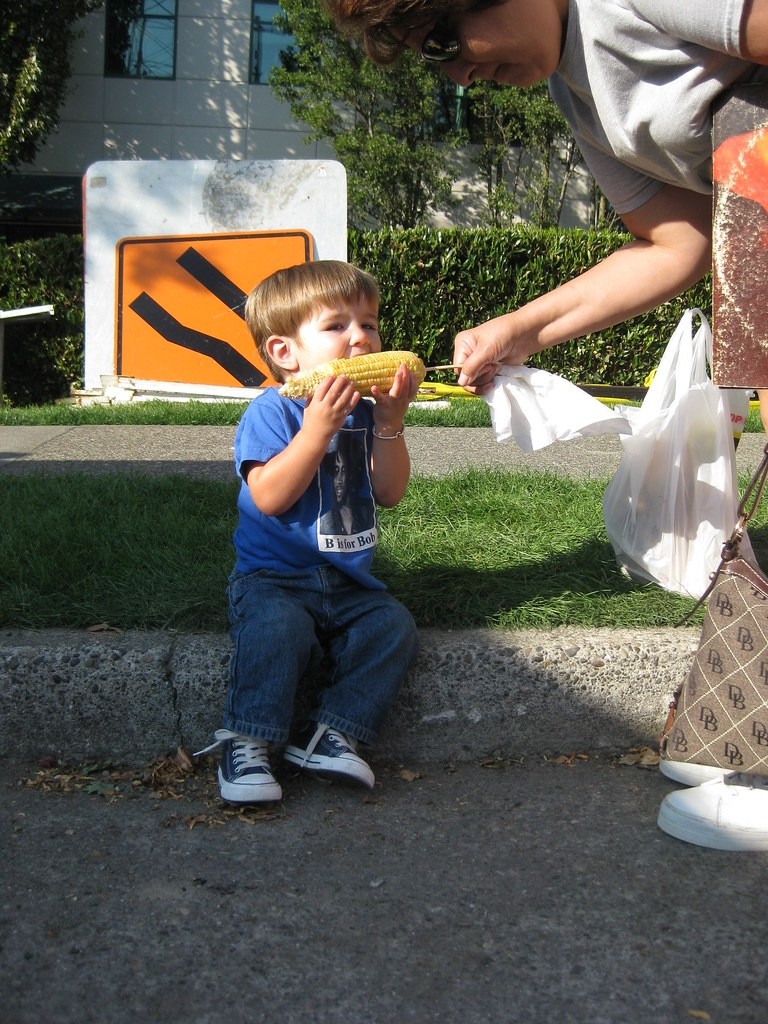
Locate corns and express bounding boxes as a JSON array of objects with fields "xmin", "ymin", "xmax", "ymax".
[{"xmin": 277, "ymin": 351, "xmax": 427, "ymax": 401}]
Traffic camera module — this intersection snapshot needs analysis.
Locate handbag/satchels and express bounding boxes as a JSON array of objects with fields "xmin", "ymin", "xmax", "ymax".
[{"xmin": 602, "ymin": 308, "xmax": 761, "ymax": 602}]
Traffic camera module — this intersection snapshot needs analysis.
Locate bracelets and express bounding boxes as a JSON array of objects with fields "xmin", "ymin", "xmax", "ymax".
[{"xmin": 372, "ymin": 423, "xmax": 405, "ymax": 440}]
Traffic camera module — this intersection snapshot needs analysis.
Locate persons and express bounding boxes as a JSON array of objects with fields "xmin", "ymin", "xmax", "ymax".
[
  {"xmin": 216, "ymin": 260, "xmax": 417, "ymax": 802},
  {"xmin": 329, "ymin": 0, "xmax": 768, "ymax": 852}
]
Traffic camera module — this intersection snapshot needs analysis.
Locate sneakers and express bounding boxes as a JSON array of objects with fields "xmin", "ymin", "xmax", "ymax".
[
  {"xmin": 192, "ymin": 727, "xmax": 282, "ymax": 801},
  {"xmin": 657, "ymin": 771, "xmax": 768, "ymax": 851},
  {"xmin": 659, "ymin": 758, "xmax": 736, "ymax": 786},
  {"xmin": 283, "ymin": 720, "xmax": 374, "ymax": 790}
]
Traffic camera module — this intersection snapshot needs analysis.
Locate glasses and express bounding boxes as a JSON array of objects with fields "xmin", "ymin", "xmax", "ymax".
[{"xmin": 418, "ymin": 6, "xmax": 461, "ymax": 62}]
